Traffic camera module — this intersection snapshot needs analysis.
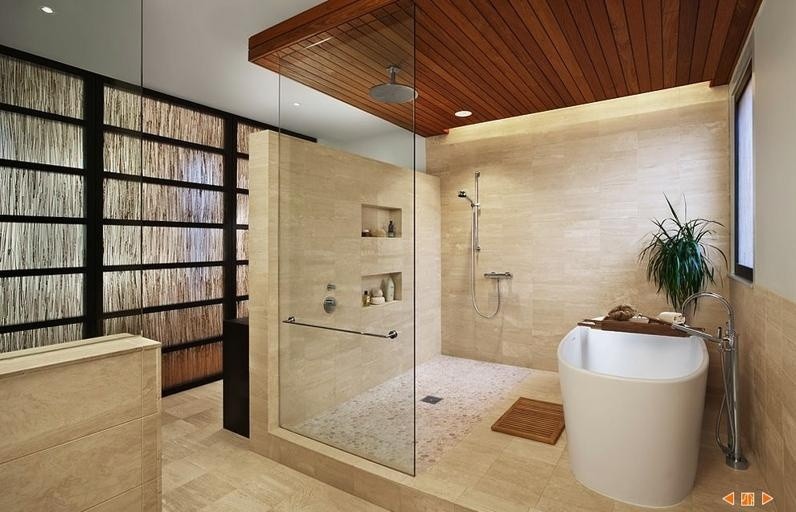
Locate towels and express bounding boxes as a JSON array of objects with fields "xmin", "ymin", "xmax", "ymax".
[{"xmin": 658, "ymin": 311, "xmax": 686, "ymax": 325}]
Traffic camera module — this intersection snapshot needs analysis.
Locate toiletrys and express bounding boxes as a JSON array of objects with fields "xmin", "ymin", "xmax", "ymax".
[
  {"xmin": 363, "ymin": 229, "xmax": 370, "ymax": 232},
  {"xmin": 388, "ymin": 221, "xmax": 395, "ymax": 237},
  {"xmin": 385, "ymin": 275, "xmax": 395, "ymax": 302}
]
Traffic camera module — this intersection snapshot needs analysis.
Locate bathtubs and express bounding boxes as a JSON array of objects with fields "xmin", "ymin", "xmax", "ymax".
[{"xmin": 557, "ymin": 315, "xmax": 710, "ymax": 508}]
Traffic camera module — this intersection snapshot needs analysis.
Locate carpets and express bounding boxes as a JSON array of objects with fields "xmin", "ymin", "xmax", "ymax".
[{"xmin": 291, "ymin": 354, "xmax": 535, "ymax": 478}]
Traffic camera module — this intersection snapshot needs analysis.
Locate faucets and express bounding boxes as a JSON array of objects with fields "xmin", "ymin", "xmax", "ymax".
[{"xmin": 681, "ymin": 290, "xmax": 734, "ymax": 335}]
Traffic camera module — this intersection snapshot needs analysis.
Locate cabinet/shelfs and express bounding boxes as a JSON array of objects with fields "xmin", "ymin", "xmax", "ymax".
[{"xmin": 0, "ymin": 43, "xmax": 317, "ymax": 398}]
